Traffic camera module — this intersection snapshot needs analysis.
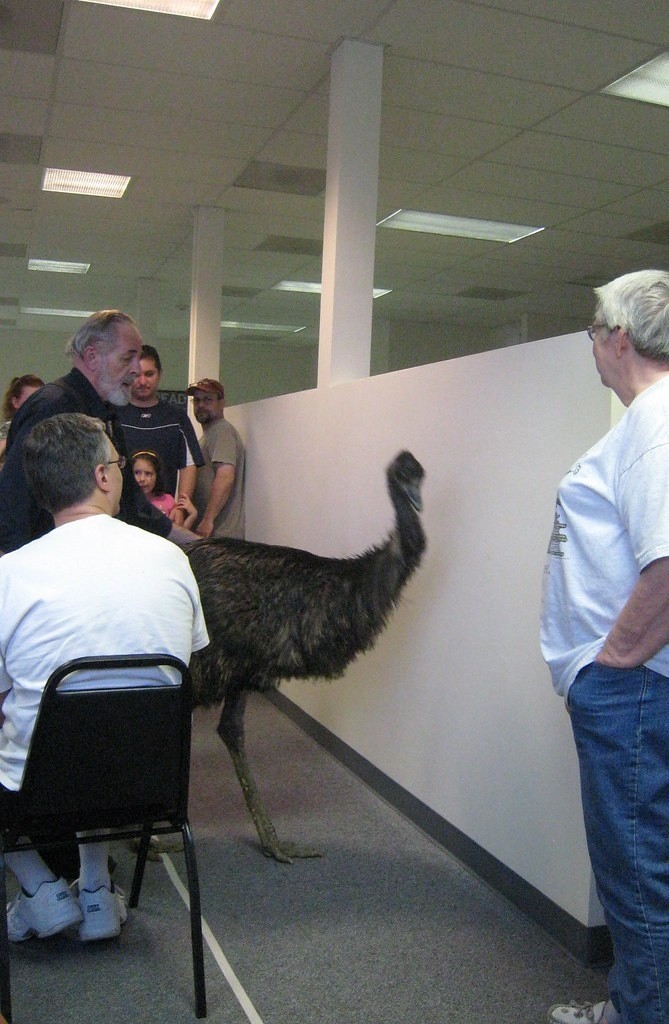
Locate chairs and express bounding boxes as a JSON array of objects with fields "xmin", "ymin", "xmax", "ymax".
[{"xmin": 0, "ymin": 653, "xmax": 207, "ymax": 1023}]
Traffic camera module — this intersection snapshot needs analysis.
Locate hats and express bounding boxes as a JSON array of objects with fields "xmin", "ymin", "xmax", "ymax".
[{"xmin": 186, "ymin": 378, "xmax": 224, "ymax": 399}]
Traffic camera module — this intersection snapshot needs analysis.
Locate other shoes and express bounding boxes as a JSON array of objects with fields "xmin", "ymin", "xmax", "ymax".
[{"xmin": 545, "ymin": 997, "xmax": 606, "ymax": 1024}]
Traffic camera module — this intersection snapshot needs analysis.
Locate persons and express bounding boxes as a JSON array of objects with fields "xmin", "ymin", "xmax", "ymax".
[
  {"xmin": 540, "ymin": 269, "xmax": 669, "ymax": 1024},
  {"xmin": 119, "ymin": 345, "xmax": 206, "ymax": 528},
  {"xmin": 187, "ymin": 378, "xmax": 246, "ymax": 540},
  {"xmin": 0, "ymin": 414, "xmax": 211, "ymax": 943},
  {"xmin": 0, "ymin": 309, "xmax": 204, "ymax": 556},
  {"xmin": 0, "ymin": 375, "xmax": 45, "ymax": 469}
]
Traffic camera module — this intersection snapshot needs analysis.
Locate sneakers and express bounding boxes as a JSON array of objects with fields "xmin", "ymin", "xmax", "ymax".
[
  {"xmin": 67, "ymin": 878, "xmax": 128, "ymax": 940},
  {"xmin": 6, "ymin": 878, "xmax": 84, "ymax": 942}
]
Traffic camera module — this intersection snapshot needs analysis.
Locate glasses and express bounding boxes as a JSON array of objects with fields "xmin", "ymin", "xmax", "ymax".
[
  {"xmin": 587, "ymin": 324, "xmax": 610, "ymax": 341},
  {"xmin": 190, "ymin": 396, "xmax": 220, "ymax": 405}
]
